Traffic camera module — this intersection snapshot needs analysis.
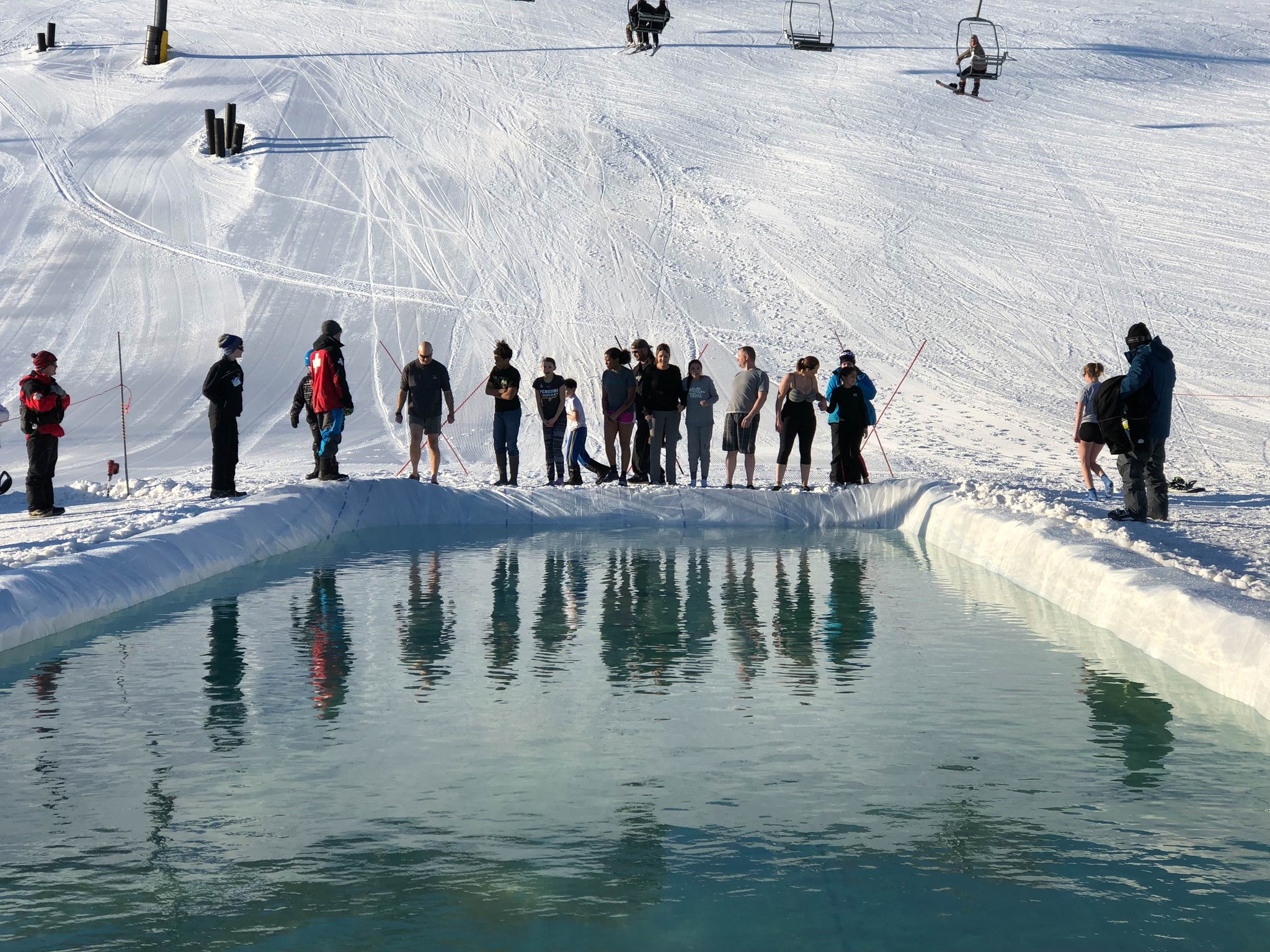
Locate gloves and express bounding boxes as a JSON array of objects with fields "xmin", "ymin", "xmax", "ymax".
[
  {"xmin": 344, "ymin": 400, "xmax": 354, "ymax": 416},
  {"xmin": 853, "ymin": 366, "xmax": 863, "ymax": 376},
  {"xmin": 291, "ymin": 416, "xmax": 299, "ymax": 428}
]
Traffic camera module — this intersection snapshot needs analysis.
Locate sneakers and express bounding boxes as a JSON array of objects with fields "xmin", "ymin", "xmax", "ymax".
[
  {"xmin": 1108, "ymin": 506, "xmax": 1147, "ymax": 523},
  {"xmin": 1146, "ymin": 512, "xmax": 1168, "ymax": 521}
]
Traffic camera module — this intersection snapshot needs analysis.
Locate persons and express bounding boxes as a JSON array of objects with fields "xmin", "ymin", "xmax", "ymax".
[
  {"xmin": 953, "ymin": 34, "xmax": 987, "ymax": 97},
  {"xmin": 1098, "ymin": 321, "xmax": 1177, "ymax": 523},
  {"xmin": 625, "ymin": 0, "xmax": 670, "ymax": 48},
  {"xmin": 563, "ymin": 378, "xmax": 610, "ymax": 485},
  {"xmin": 678, "ymin": 359, "xmax": 718, "ymax": 488},
  {"xmin": 310, "ymin": 319, "xmax": 354, "ymax": 482},
  {"xmin": 203, "ymin": 333, "xmax": 247, "ymax": 498},
  {"xmin": 290, "ymin": 349, "xmax": 323, "ymax": 480},
  {"xmin": 818, "ymin": 350, "xmax": 877, "ymax": 486},
  {"xmin": 0, "ymin": 405, "xmax": 9, "ymax": 426},
  {"xmin": 18, "ymin": 350, "xmax": 70, "ymax": 518},
  {"xmin": 485, "ymin": 342, "xmax": 521, "ymax": 486},
  {"xmin": 721, "ymin": 346, "xmax": 769, "ymax": 490},
  {"xmin": 532, "ymin": 358, "xmax": 565, "ymax": 486},
  {"xmin": 1073, "ymin": 363, "xmax": 1114, "ymax": 503},
  {"xmin": 602, "ymin": 338, "xmax": 666, "ymax": 485},
  {"xmin": 642, "ymin": 343, "xmax": 686, "ymax": 485},
  {"xmin": 770, "ymin": 356, "xmax": 827, "ymax": 491},
  {"xmin": 395, "ymin": 341, "xmax": 455, "ymax": 485}
]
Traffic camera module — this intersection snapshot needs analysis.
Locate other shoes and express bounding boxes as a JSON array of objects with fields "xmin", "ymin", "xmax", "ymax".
[
  {"xmin": 29, "ymin": 509, "xmax": 53, "ymax": 517},
  {"xmin": 210, "ymin": 489, "xmax": 235, "ymax": 498},
  {"xmin": 659, "ymin": 475, "xmax": 666, "ymax": 485},
  {"xmin": 627, "ymin": 474, "xmax": 649, "ymax": 484},
  {"xmin": 48, "ymin": 506, "xmax": 65, "ymax": 516},
  {"xmin": 227, "ymin": 489, "xmax": 247, "ymax": 497},
  {"xmin": 846, "ymin": 482, "xmax": 851, "ymax": 487},
  {"xmin": 830, "ymin": 481, "xmax": 844, "ymax": 487}
]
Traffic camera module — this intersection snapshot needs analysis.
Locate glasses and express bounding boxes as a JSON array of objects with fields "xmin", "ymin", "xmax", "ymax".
[
  {"xmin": 1083, "ymin": 372, "xmax": 1088, "ymax": 378},
  {"xmin": 237, "ymin": 346, "xmax": 243, "ymax": 351},
  {"xmin": 631, "ymin": 349, "xmax": 643, "ymax": 354},
  {"xmin": 418, "ymin": 350, "xmax": 433, "ymax": 358},
  {"xmin": 50, "ymin": 362, "xmax": 57, "ymax": 366}
]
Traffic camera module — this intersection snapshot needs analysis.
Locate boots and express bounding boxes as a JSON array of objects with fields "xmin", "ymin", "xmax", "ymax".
[
  {"xmin": 586, "ymin": 458, "xmax": 610, "ymax": 485},
  {"xmin": 305, "ymin": 459, "xmax": 349, "ymax": 481},
  {"xmin": 563, "ymin": 466, "xmax": 583, "ymax": 485}
]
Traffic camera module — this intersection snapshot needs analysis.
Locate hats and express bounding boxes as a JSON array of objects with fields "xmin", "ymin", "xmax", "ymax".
[
  {"xmin": 1125, "ymin": 322, "xmax": 1152, "ymax": 351},
  {"xmin": 839, "ymin": 350, "xmax": 855, "ymax": 365},
  {"xmin": 631, "ymin": 339, "xmax": 654, "ymax": 349},
  {"xmin": 31, "ymin": 350, "xmax": 57, "ymax": 370},
  {"xmin": 322, "ymin": 320, "xmax": 341, "ymax": 336},
  {"xmin": 218, "ymin": 333, "xmax": 242, "ymax": 356}
]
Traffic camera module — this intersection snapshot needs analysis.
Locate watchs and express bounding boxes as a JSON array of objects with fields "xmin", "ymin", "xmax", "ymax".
[{"xmin": 395, "ymin": 412, "xmax": 401, "ymax": 416}]
